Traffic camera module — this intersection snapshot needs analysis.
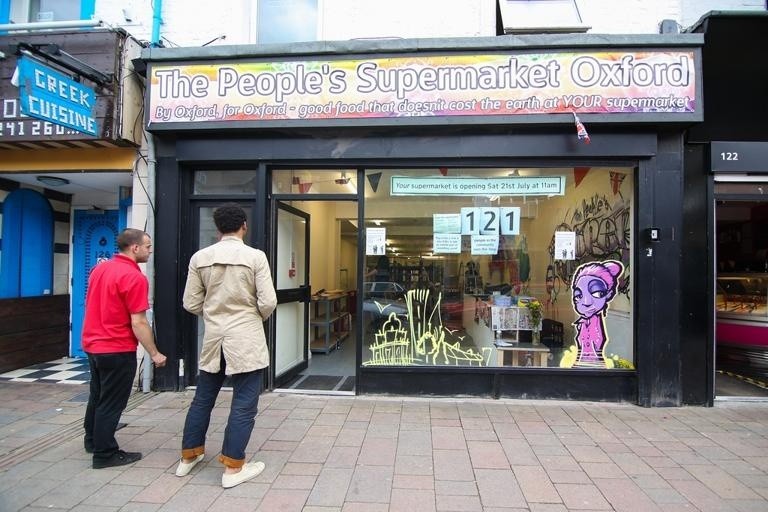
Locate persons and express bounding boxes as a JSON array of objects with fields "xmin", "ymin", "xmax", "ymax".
[
  {"xmin": 82, "ymin": 226, "xmax": 168, "ymax": 470},
  {"xmin": 363, "ymin": 255, "xmax": 390, "ymax": 334},
  {"xmin": 174, "ymin": 204, "xmax": 280, "ymax": 491},
  {"xmin": 465, "ymin": 262, "xmax": 479, "ymax": 293},
  {"xmin": 545, "ymin": 263, "xmax": 559, "ymax": 322}
]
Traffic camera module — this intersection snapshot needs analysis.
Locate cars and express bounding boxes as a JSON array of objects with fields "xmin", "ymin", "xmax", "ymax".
[{"xmin": 363, "ymin": 279, "xmax": 496, "ymax": 337}]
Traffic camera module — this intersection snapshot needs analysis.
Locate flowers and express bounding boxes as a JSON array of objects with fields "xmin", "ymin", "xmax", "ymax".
[{"xmin": 526, "ymin": 294, "xmax": 544, "ymax": 328}]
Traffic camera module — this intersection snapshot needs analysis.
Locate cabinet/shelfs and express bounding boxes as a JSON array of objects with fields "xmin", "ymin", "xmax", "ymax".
[{"xmin": 310, "ymin": 292, "xmax": 352, "ymax": 355}]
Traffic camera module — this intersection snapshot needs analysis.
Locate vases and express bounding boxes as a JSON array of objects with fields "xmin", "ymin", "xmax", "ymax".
[{"xmin": 531, "ymin": 326, "xmax": 541, "ymax": 347}]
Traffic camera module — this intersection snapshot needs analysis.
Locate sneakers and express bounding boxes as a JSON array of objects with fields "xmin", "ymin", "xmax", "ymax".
[
  {"xmin": 175, "ymin": 452, "xmax": 205, "ymax": 478},
  {"xmin": 85, "ymin": 434, "xmax": 142, "ymax": 469},
  {"xmin": 221, "ymin": 460, "xmax": 266, "ymax": 489}
]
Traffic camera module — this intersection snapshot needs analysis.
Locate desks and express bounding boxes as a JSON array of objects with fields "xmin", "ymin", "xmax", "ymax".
[{"xmin": 363, "ymin": 297, "xmax": 410, "ymax": 338}]
